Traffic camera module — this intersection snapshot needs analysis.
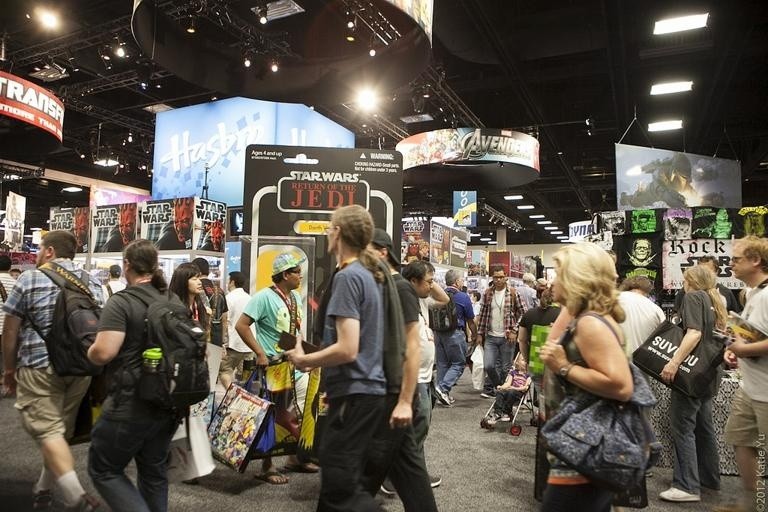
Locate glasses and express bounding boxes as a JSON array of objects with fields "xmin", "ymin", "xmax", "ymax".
[
  {"xmin": 287, "ymin": 269, "xmax": 302, "ymax": 276},
  {"xmin": 492, "ymin": 275, "xmax": 506, "ymax": 280},
  {"xmin": 323, "ymin": 227, "xmax": 337, "ymax": 234},
  {"xmin": 730, "ymin": 255, "xmax": 748, "ymax": 264}
]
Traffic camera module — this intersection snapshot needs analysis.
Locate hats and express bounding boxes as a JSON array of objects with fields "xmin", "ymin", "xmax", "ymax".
[
  {"xmin": 272, "ymin": 253, "xmax": 307, "ymax": 278},
  {"xmin": 537, "ymin": 278, "xmax": 548, "ymax": 286},
  {"xmin": 370, "ymin": 228, "xmax": 402, "ymax": 270},
  {"xmin": 522, "ymin": 272, "xmax": 536, "ymax": 283}
]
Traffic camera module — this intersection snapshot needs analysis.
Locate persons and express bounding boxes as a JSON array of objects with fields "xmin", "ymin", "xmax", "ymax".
[
  {"xmin": 0, "ymin": 204, "xmax": 768, "ymax": 512},
  {"xmin": 442, "ymin": 132, "xmax": 462, "ymax": 161}
]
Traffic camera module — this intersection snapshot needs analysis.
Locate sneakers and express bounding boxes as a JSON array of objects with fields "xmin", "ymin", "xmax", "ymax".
[
  {"xmin": 436, "ymin": 385, "xmax": 457, "ymax": 408},
  {"xmin": 427, "ymin": 473, "xmax": 442, "ymax": 488},
  {"xmin": 481, "ymin": 391, "xmax": 498, "ymax": 398},
  {"xmin": 486, "ymin": 413, "xmax": 511, "ymax": 427},
  {"xmin": 381, "ymin": 474, "xmax": 397, "ymax": 495},
  {"xmin": 658, "ymin": 485, "xmax": 703, "ymax": 503},
  {"xmin": 54, "ymin": 493, "xmax": 102, "ymax": 511},
  {"xmin": 28, "ymin": 485, "xmax": 53, "ymax": 512}
]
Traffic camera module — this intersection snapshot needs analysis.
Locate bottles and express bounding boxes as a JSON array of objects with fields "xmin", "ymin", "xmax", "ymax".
[{"xmin": 142, "ymin": 347, "xmax": 163, "ymax": 376}]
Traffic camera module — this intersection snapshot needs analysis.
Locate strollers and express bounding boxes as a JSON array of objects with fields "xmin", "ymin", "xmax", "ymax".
[{"xmin": 478, "ymin": 346, "xmax": 538, "ymax": 437}]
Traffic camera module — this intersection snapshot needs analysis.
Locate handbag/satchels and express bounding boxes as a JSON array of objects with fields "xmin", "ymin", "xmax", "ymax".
[
  {"xmin": 533, "ymin": 418, "xmax": 649, "ymax": 509},
  {"xmin": 165, "ymin": 365, "xmax": 277, "ymax": 485},
  {"xmin": 243, "ymin": 355, "xmax": 306, "ymax": 461},
  {"xmin": 538, "ymin": 313, "xmax": 662, "ymax": 492},
  {"xmin": 631, "ymin": 291, "xmax": 726, "ymax": 400}
]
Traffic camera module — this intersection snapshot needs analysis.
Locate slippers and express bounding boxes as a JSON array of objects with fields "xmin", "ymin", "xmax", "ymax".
[
  {"xmin": 285, "ymin": 463, "xmax": 320, "ymax": 473},
  {"xmin": 253, "ymin": 469, "xmax": 290, "ymax": 486}
]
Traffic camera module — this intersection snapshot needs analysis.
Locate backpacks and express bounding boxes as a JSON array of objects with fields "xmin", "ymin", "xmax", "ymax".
[
  {"xmin": 428, "ymin": 289, "xmax": 459, "ymax": 333},
  {"xmin": 119, "ymin": 288, "xmax": 211, "ymax": 419},
  {"xmin": 30, "ymin": 268, "xmax": 105, "ymax": 378}
]
